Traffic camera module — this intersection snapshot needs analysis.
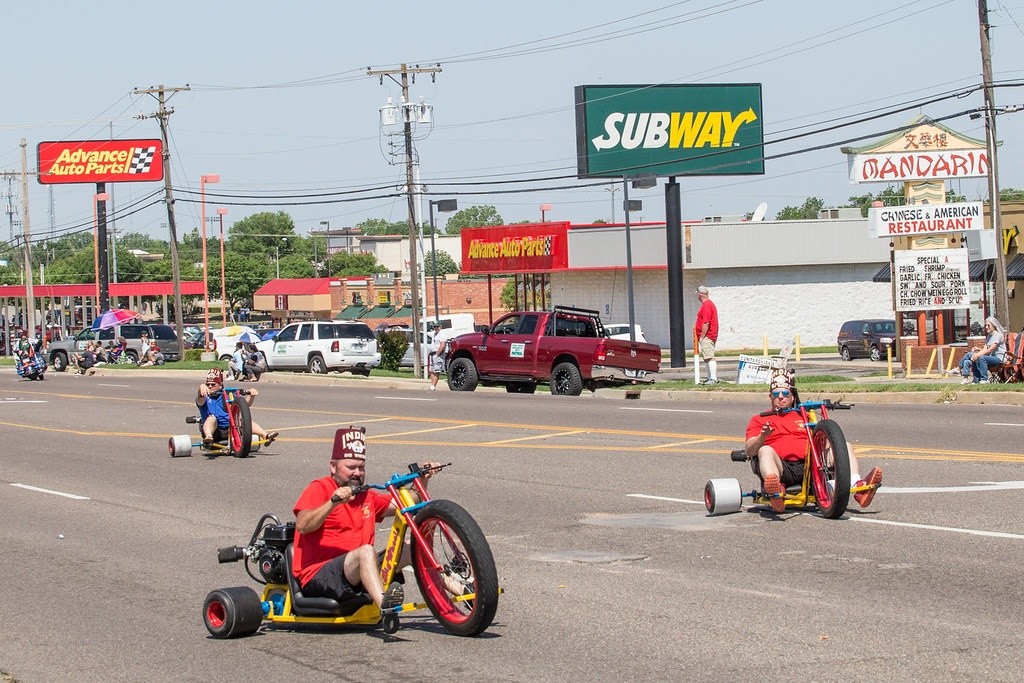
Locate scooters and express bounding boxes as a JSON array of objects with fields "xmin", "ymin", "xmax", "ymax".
[{"xmin": 13, "ymin": 338, "xmax": 49, "ymax": 381}]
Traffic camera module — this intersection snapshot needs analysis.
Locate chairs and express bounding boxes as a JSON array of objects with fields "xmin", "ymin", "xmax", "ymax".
[
  {"xmin": 284, "ymin": 542, "xmax": 372, "ymax": 616},
  {"xmin": 987, "ymin": 331, "xmax": 1024, "ymax": 383}
]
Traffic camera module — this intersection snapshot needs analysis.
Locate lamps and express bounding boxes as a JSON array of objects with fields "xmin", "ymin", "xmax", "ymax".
[{"xmin": 466, "ymin": 298, "xmax": 471, "ymax": 304}]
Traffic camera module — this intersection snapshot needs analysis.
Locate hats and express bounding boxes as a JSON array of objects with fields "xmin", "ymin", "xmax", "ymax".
[
  {"xmin": 332, "ymin": 425, "xmax": 366, "ymax": 460},
  {"xmin": 154, "ymin": 347, "xmax": 160, "ymax": 350},
  {"xmin": 695, "ymin": 286, "xmax": 709, "ymax": 294},
  {"xmin": 770, "ymin": 368, "xmax": 795, "ymax": 395},
  {"xmin": 206, "ymin": 368, "xmax": 222, "ymax": 384},
  {"xmin": 432, "ymin": 321, "xmax": 441, "ymax": 327}
]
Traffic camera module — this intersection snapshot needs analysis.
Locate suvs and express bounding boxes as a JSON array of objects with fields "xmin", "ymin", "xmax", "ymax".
[
  {"xmin": 836, "ymin": 318, "xmax": 905, "ymax": 362},
  {"xmin": 45, "ymin": 320, "xmax": 180, "ymax": 373},
  {"xmin": 250, "ymin": 318, "xmax": 383, "ymax": 375}
]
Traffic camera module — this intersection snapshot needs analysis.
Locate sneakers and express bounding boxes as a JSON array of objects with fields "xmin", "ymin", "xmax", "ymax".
[
  {"xmin": 764, "ymin": 473, "xmax": 786, "ymax": 512},
  {"xmin": 852, "ymin": 467, "xmax": 883, "ymax": 508}
]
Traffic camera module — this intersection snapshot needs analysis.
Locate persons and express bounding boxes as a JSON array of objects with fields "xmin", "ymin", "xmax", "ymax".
[
  {"xmin": 257, "ymin": 322, "xmax": 270, "ymax": 334},
  {"xmin": 243, "ymin": 345, "xmax": 266, "ymax": 381},
  {"xmin": 224, "ymin": 342, "xmax": 247, "ymax": 380},
  {"xmin": 72, "ymin": 333, "xmax": 164, "ymax": 375},
  {"xmin": 227, "ymin": 305, "xmax": 251, "ymax": 322},
  {"xmin": 744, "ymin": 368, "xmax": 883, "ymax": 512},
  {"xmin": 427, "ymin": 324, "xmax": 447, "ymax": 391},
  {"xmin": 694, "ymin": 286, "xmax": 719, "ymax": 385},
  {"xmin": 13, "ymin": 334, "xmax": 42, "ymax": 357},
  {"xmin": 292, "ymin": 425, "xmax": 441, "ymax": 615},
  {"xmin": 943, "ymin": 317, "xmax": 1008, "ymax": 385},
  {"xmin": 195, "ymin": 368, "xmax": 279, "ymax": 449}
]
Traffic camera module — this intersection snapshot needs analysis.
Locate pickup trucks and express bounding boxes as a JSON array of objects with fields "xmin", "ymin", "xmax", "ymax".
[{"xmin": 443, "ymin": 303, "xmax": 663, "ymax": 397}]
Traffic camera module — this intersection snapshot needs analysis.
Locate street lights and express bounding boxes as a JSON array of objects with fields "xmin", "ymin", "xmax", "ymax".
[
  {"xmin": 622, "ymin": 173, "xmax": 657, "ymax": 343},
  {"xmin": 320, "ymin": 221, "xmax": 331, "ymax": 279},
  {"xmin": 429, "ymin": 198, "xmax": 458, "ymax": 322},
  {"xmin": 276, "ymin": 237, "xmax": 288, "ymax": 278},
  {"xmin": 200, "ymin": 173, "xmax": 221, "ymax": 352},
  {"xmin": 217, "ymin": 207, "xmax": 228, "ymax": 328}
]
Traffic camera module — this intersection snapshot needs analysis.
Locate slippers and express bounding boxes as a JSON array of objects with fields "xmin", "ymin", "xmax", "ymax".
[
  {"xmin": 203, "ymin": 437, "xmax": 213, "ymax": 449},
  {"xmin": 264, "ymin": 432, "xmax": 278, "ymax": 447}
]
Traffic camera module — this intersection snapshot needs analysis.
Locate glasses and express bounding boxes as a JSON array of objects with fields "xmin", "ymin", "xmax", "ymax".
[
  {"xmin": 206, "ymin": 383, "xmax": 216, "ymax": 386},
  {"xmin": 772, "ymin": 390, "xmax": 790, "ymax": 397}
]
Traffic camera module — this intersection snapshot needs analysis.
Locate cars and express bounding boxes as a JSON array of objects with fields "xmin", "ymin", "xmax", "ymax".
[
  {"xmin": 372, "ymin": 326, "xmax": 448, "ymax": 374},
  {"xmin": 165, "ymin": 321, "xmax": 262, "ymax": 366}
]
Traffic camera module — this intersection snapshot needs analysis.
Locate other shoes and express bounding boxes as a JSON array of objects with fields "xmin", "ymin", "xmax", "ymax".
[
  {"xmin": 947, "ymin": 370, "xmax": 961, "ymax": 377},
  {"xmin": 961, "ymin": 380, "xmax": 970, "ymax": 384},
  {"xmin": 428, "ymin": 389, "xmax": 436, "ymax": 392},
  {"xmin": 703, "ymin": 379, "xmax": 720, "ymax": 386},
  {"xmin": 244, "ymin": 379, "xmax": 249, "ymax": 382},
  {"xmin": 74, "ymin": 370, "xmax": 83, "ymax": 375},
  {"xmin": 980, "ymin": 381, "xmax": 990, "ymax": 384},
  {"xmin": 464, "ymin": 580, "xmax": 476, "ymax": 611},
  {"xmin": 226, "ymin": 376, "xmax": 235, "ymax": 380},
  {"xmin": 249, "ymin": 378, "xmax": 258, "ymax": 381},
  {"xmin": 237, "ymin": 374, "xmax": 245, "ymax": 381},
  {"xmin": 137, "ymin": 361, "xmax": 142, "ymax": 366},
  {"xmin": 699, "ymin": 379, "xmax": 709, "ymax": 384},
  {"xmin": 380, "ymin": 581, "xmax": 404, "ymax": 634}
]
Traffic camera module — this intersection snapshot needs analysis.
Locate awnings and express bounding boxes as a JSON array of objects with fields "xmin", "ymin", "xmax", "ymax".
[
  {"xmin": 873, "ymin": 262, "xmax": 891, "ymax": 282},
  {"xmin": 968, "ymin": 259, "xmax": 994, "ymax": 282},
  {"xmin": 1006, "ymin": 253, "xmax": 1024, "ymax": 281}
]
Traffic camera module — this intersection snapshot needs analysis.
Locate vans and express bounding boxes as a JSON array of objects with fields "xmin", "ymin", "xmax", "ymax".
[{"xmin": 409, "ymin": 311, "xmax": 476, "ymax": 344}]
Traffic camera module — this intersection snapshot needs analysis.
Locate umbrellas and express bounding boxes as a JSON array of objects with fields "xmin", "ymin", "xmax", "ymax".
[
  {"xmin": 216, "ymin": 325, "xmax": 292, "ymax": 343},
  {"xmin": 89, "ymin": 307, "xmax": 141, "ymax": 341}
]
